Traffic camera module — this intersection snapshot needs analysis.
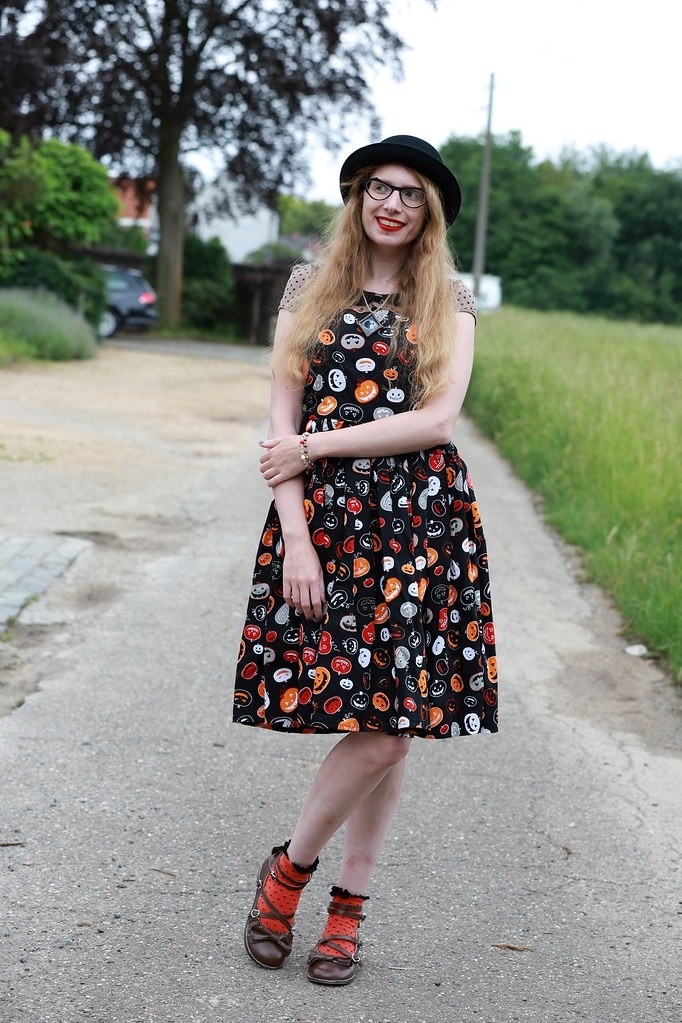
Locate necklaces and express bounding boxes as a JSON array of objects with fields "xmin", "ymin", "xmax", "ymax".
[{"xmin": 356, "ymin": 286, "xmax": 393, "ymax": 337}]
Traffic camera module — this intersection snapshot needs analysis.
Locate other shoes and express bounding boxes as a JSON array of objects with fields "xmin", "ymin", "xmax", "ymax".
[
  {"xmin": 305, "ymin": 901, "xmax": 368, "ymax": 985},
  {"xmin": 243, "ymin": 849, "xmax": 296, "ymax": 969}
]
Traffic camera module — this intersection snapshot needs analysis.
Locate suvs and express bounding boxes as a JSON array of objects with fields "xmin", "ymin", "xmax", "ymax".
[{"xmin": 89, "ymin": 265, "xmax": 161, "ymax": 338}]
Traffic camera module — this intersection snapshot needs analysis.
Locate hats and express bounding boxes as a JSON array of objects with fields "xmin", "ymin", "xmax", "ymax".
[{"xmin": 339, "ymin": 134, "xmax": 463, "ymax": 231}]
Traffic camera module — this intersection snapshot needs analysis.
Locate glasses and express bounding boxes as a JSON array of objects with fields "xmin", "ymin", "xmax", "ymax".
[{"xmin": 365, "ymin": 178, "xmax": 430, "ymax": 208}]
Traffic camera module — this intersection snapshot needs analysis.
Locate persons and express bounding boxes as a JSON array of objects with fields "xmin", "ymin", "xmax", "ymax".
[{"xmin": 231, "ymin": 135, "xmax": 499, "ymax": 987}]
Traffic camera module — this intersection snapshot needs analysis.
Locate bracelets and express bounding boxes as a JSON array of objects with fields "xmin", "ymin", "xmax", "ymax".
[{"xmin": 299, "ymin": 432, "xmax": 314, "ymax": 472}]
